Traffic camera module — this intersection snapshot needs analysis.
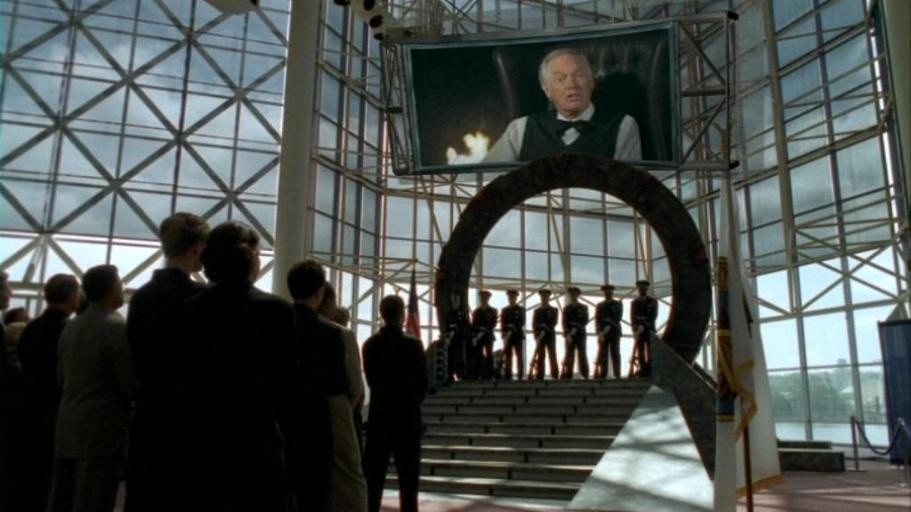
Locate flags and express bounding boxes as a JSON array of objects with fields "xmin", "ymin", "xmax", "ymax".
[
  {"xmin": 406, "ymin": 265, "xmax": 420, "ymax": 337},
  {"xmin": 713, "ymin": 133, "xmax": 784, "ymax": 511}
]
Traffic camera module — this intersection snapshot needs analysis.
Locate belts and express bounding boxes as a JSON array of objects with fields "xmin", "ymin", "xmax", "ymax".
[{"xmin": 478, "ymin": 316, "xmax": 652, "ymax": 330}]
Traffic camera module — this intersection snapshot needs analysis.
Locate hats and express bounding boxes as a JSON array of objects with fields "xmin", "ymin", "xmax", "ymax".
[{"xmin": 478, "ymin": 281, "xmax": 649, "ymax": 297}]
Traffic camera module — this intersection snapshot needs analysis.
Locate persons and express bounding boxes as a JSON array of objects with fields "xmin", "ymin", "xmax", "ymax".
[
  {"xmin": 595, "ymin": 286, "xmax": 622, "ymax": 379},
  {"xmin": 533, "ymin": 288, "xmax": 559, "ymax": 382},
  {"xmin": 362, "ymin": 295, "xmax": 427, "ymax": 512},
  {"xmin": 443, "ymin": 289, "xmax": 469, "ymax": 383},
  {"xmin": 562, "ymin": 287, "xmax": 589, "ymax": 381},
  {"xmin": 1, "ymin": 211, "xmax": 369, "ymax": 511},
  {"xmin": 472, "ymin": 291, "xmax": 496, "ymax": 384},
  {"xmin": 479, "ymin": 49, "xmax": 644, "ymax": 163},
  {"xmin": 501, "ymin": 290, "xmax": 525, "ymax": 381},
  {"xmin": 629, "ymin": 280, "xmax": 658, "ymax": 374}
]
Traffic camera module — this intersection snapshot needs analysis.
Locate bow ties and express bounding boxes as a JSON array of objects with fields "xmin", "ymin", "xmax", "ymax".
[{"xmin": 554, "ymin": 119, "xmax": 588, "ymax": 134}]
